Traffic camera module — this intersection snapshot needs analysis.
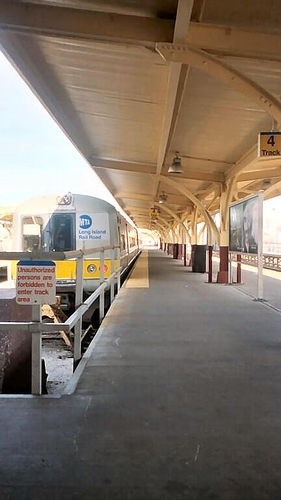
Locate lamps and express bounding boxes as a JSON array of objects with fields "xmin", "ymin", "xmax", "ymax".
[
  {"xmin": 149, "ymin": 185, "xmax": 167, "ymax": 230},
  {"xmin": 166, "ymin": 151, "xmax": 184, "ymax": 174}
]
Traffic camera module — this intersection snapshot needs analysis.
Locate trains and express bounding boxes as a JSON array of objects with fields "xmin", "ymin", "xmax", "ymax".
[{"xmin": 7, "ymin": 191, "xmax": 143, "ymax": 293}]
